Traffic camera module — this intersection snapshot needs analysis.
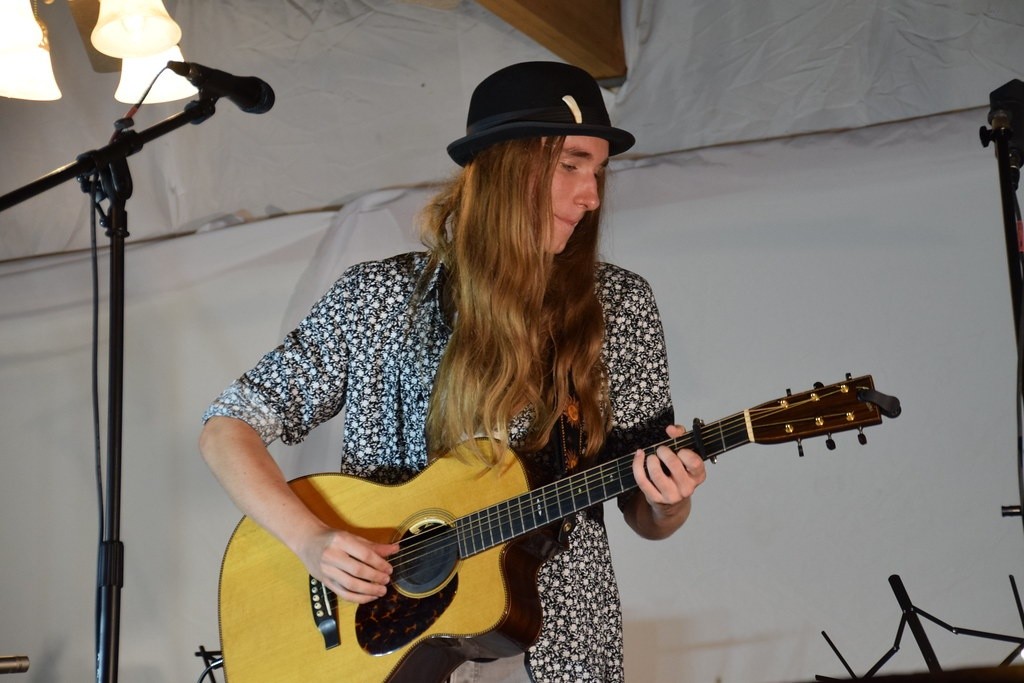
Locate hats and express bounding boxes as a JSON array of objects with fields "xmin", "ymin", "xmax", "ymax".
[{"xmin": 447, "ymin": 60, "xmax": 636, "ymax": 166}]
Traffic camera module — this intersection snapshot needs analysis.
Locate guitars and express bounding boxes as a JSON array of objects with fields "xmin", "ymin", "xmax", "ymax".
[{"xmin": 218, "ymin": 373, "xmax": 905, "ymax": 683}]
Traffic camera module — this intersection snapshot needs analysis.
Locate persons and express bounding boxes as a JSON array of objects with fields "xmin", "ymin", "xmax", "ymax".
[{"xmin": 197, "ymin": 59, "xmax": 706, "ymax": 683}]
